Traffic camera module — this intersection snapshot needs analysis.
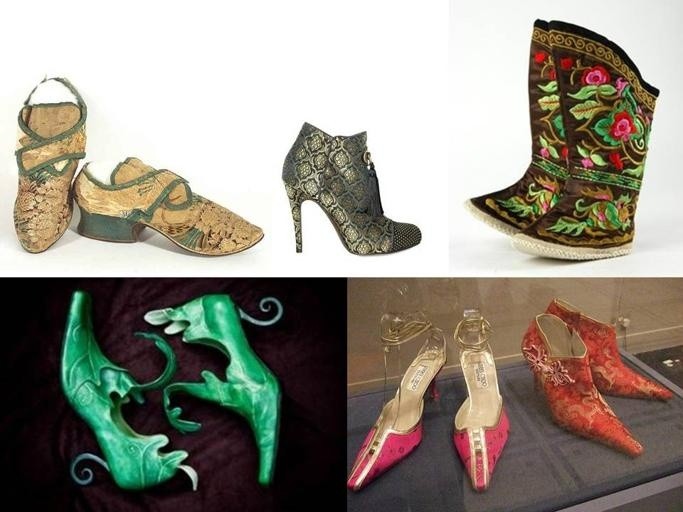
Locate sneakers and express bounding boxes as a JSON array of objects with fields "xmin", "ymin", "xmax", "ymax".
[
  {"xmin": 13, "ymin": 76, "xmax": 86, "ymax": 253},
  {"xmin": 71, "ymin": 157, "xmax": 265, "ymax": 254}
]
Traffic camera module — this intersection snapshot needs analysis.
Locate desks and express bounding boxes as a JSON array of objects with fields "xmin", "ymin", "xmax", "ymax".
[{"xmin": 348, "ymin": 345, "xmax": 683, "ymax": 511}]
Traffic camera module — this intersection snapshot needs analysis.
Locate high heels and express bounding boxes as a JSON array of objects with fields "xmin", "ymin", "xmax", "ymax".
[
  {"xmin": 143, "ymin": 296, "xmax": 284, "ymax": 486},
  {"xmin": 61, "ymin": 290, "xmax": 199, "ymax": 493},
  {"xmin": 347, "ymin": 320, "xmax": 448, "ymax": 491},
  {"xmin": 521, "ymin": 314, "xmax": 646, "ymax": 458},
  {"xmin": 543, "ymin": 297, "xmax": 673, "ymax": 401},
  {"xmin": 452, "ymin": 317, "xmax": 510, "ymax": 493},
  {"xmin": 281, "ymin": 122, "xmax": 422, "ymax": 256}
]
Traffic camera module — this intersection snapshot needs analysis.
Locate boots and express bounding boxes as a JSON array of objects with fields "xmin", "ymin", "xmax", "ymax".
[
  {"xmin": 463, "ymin": 20, "xmax": 567, "ymax": 236},
  {"xmin": 515, "ymin": 24, "xmax": 660, "ymax": 259}
]
